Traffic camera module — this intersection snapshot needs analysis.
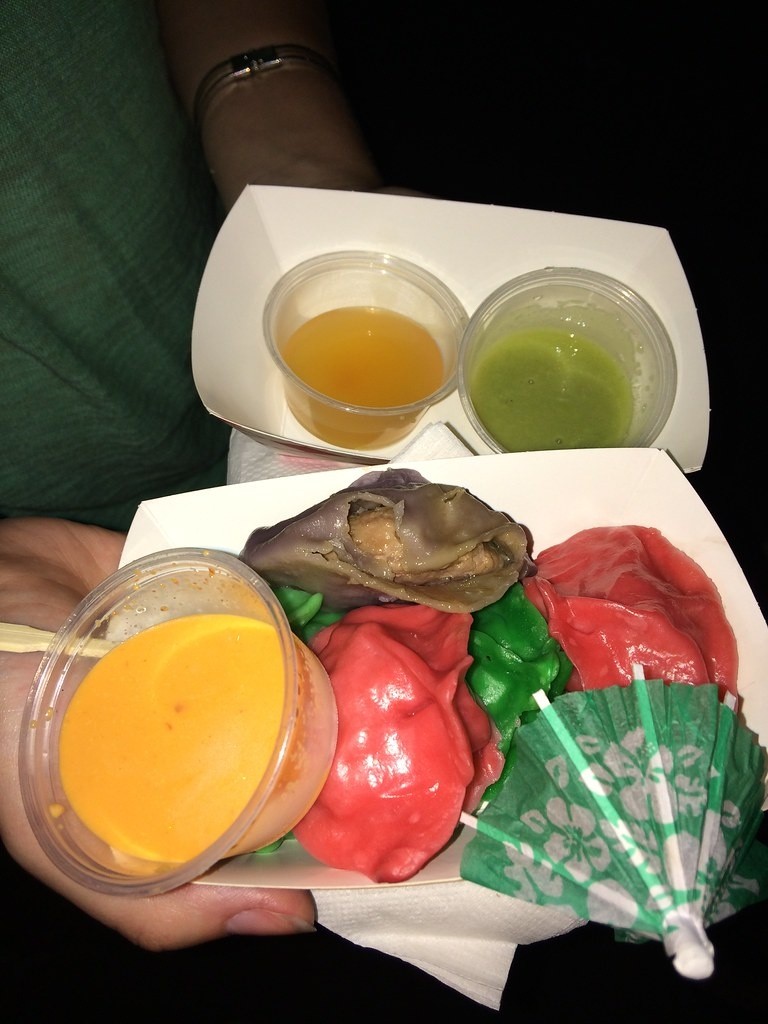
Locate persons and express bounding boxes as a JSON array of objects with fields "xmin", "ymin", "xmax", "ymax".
[{"xmin": 0, "ymin": 0, "xmax": 593, "ymax": 1022}]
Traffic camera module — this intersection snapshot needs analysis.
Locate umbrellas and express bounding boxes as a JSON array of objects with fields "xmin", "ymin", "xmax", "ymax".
[{"xmin": 456, "ymin": 660, "xmax": 768, "ymax": 978}]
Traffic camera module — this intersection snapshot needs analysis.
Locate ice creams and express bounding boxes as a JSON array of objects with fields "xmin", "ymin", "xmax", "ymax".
[{"xmin": 238, "ymin": 470, "xmax": 741, "ymax": 884}]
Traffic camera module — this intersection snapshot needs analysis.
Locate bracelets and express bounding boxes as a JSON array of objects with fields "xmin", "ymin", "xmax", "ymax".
[{"xmin": 189, "ymin": 36, "xmax": 348, "ymax": 156}]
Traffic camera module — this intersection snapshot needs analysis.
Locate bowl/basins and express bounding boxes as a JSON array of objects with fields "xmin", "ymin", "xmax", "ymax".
[
  {"xmin": 458, "ymin": 261, "xmax": 679, "ymax": 466},
  {"xmin": 258, "ymin": 247, "xmax": 473, "ymax": 457},
  {"xmin": 19, "ymin": 542, "xmax": 338, "ymax": 900}
]
